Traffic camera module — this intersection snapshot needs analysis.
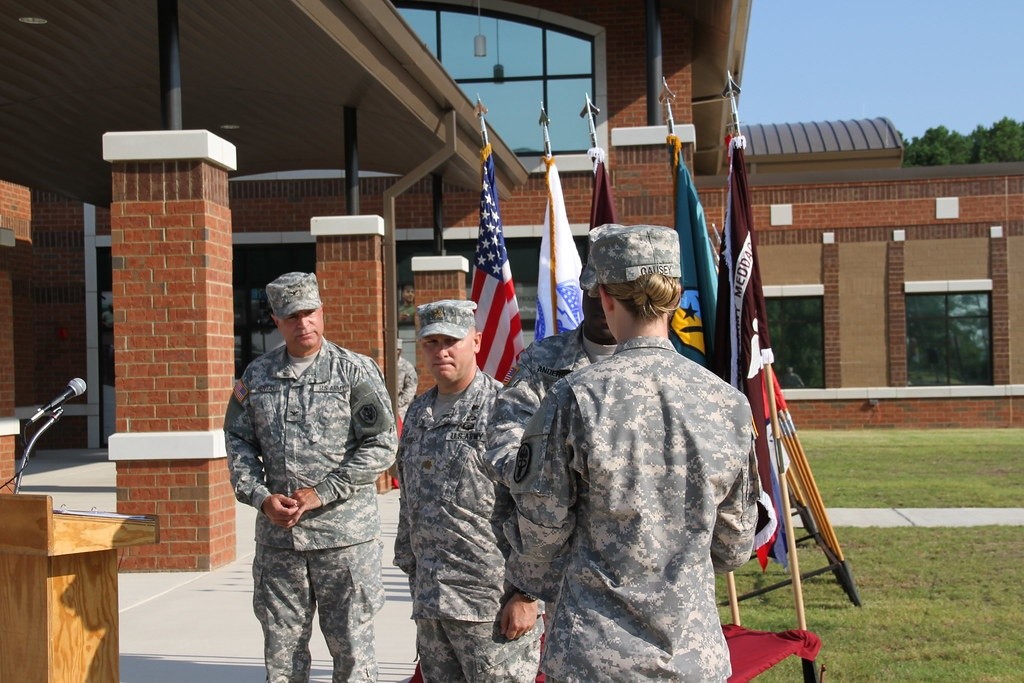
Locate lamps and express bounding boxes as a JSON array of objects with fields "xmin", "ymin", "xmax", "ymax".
[
  {"xmin": 493, "ymin": 18, "xmax": 504, "ymax": 83},
  {"xmin": 869, "ymin": 399, "xmax": 877, "ymax": 406},
  {"xmin": 475, "ymin": 0, "xmax": 486, "ymax": 57}
]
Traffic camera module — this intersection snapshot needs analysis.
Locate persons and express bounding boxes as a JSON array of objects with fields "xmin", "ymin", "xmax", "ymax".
[
  {"xmin": 397, "ymin": 338, "xmax": 418, "ymax": 424},
  {"xmin": 393, "ymin": 299, "xmax": 545, "ymax": 683},
  {"xmin": 223, "ymin": 272, "xmax": 399, "ymax": 683},
  {"xmin": 783, "ymin": 367, "xmax": 804, "ymax": 388},
  {"xmin": 510, "ymin": 224, "xmax": 763, "ymax": 683},
  {"xmin": 483, "ymin": 225, "xmax": 619, "ymax": 612}
]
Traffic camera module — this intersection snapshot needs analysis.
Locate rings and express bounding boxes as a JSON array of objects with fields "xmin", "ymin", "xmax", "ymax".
[{"xmin": 295, "ymin": 504, "xmax": 297, "ymax": 507}]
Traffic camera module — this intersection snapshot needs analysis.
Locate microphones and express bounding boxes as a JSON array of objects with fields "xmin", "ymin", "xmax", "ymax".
[{"xmin": 25, "ymin": 378, "xmax": 87, "ymax": 427}]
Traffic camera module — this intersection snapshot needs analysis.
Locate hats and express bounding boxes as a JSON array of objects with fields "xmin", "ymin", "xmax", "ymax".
[
  {"xmin": 592, "ymin": 225, "xmax": 682, "ymax": 285},
  {"xmin": 580, "ymin": 224, "xmax": 624, "ymax": 298},
  {"xmin": 417, "ymin": 299, "xmax": 478, "ymax": 339},
  {"xmin": 397, "ymin": 338, "xmax": 403, "ymax": 349},
  {"xmin": 265, "ymin": 272, "xmax": 322, "ymax": 319}
]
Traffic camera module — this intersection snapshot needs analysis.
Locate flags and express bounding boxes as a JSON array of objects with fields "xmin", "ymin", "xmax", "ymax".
[{"xmin": 471, "ymin": 133, "xmax": 786, "ymax": 573}]
